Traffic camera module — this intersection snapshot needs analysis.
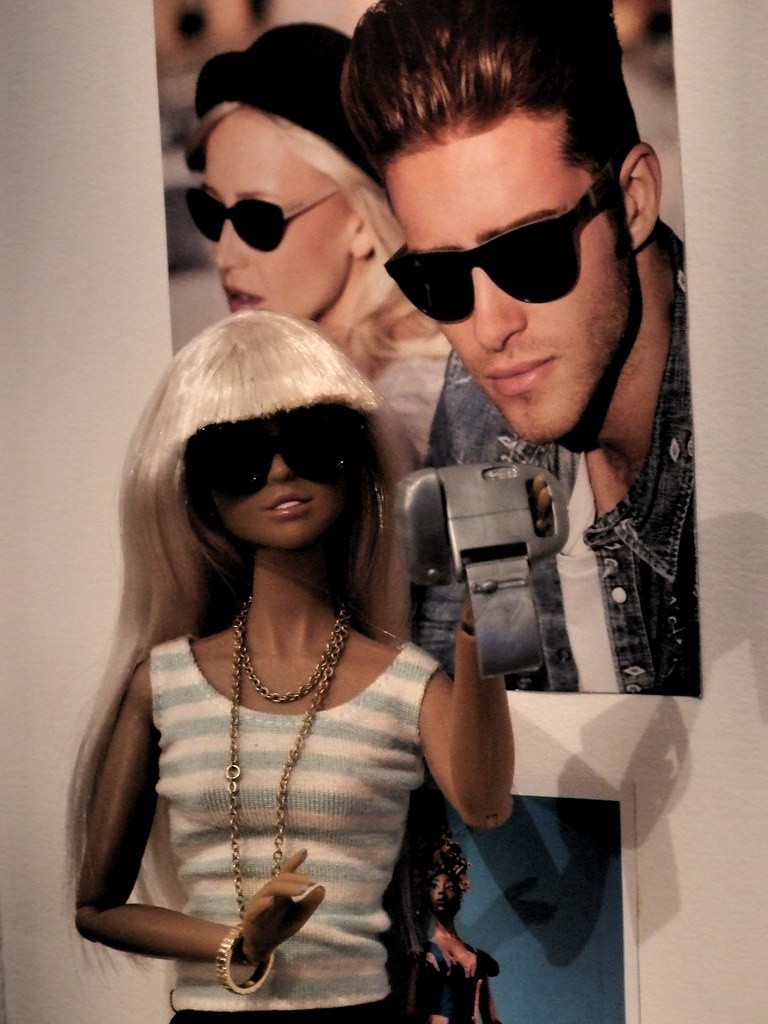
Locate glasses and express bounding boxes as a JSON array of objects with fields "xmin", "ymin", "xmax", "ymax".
[
  {"xmin": 183, "ymin": 181, "xmax": 347, "ymax": 254},
  {"xmin": 382, "ymin": 164, "xmax": 625, "ymax": 323},
  {"xmin": 180, "ymin": 401, "xmax": 376, "ymax": 506}
]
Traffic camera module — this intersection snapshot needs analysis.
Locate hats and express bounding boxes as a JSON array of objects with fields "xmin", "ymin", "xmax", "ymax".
[{"xmin": 189, "ymin": 22, "xmax": 386, "ymax": 188}]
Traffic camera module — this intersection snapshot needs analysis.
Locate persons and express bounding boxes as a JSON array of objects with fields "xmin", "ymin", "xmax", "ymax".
[
  {"xmin": 340, "ymin": 0, "xmax": 703, "ymax": 695},
  {"xmin": 387, "ymin": 869, "xmax": 500, "ymax": 1024},
  {"xmin": 64, "ymin": 307, "xmax": 552, "ymax": 1024},
  {"xmin": 178, "ymin": 22, "xmax": 452, "ymax": 455}
]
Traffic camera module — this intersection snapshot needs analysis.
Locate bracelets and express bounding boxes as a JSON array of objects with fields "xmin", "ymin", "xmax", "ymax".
[{"xmin": 214, "ymin": 924, "xmax": 275, "ymax": 995}]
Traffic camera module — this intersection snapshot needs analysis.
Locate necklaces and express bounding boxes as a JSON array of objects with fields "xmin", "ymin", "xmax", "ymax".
[{"xmin": 226, "ymin": 589, "xmax": 355, "ymax": 922}]
